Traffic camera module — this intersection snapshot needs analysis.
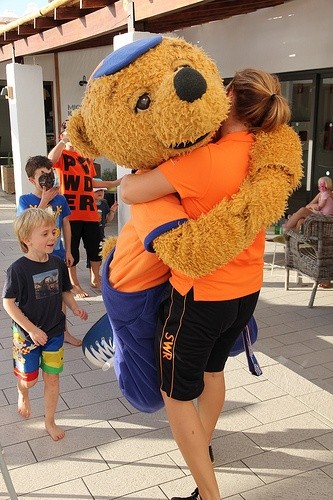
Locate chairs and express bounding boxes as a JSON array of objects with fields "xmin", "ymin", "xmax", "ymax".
[{"xmin": 284, "ymin": 213, "xmax": 333, "ymax": 307}]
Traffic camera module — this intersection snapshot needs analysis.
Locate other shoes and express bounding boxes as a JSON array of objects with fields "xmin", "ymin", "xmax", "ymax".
[
  {"xmin": 208, "ymin": 443, "xmax": 215, "ymax": 463},
  {"xmin": 171, "ymin": 487, "xmax": 202, "ymax": 500}
]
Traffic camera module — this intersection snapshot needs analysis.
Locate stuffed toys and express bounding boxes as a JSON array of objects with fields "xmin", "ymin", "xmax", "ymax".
[{"xmin": 67, "ymin": 35, "xmax": 304, "ymax": 414}]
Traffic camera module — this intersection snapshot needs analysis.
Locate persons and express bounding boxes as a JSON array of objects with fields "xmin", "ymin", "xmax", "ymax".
[
  {"xmin": 16, "ymin": 155, "xmax": 83, "ymax": 347},
  {"xmin": 119, "ymin": 66, "xmax": 290, "ymax": 500},
  {"xmin": 47, "ymin": 119, "xmax": 122, "ymax": 298},
  {"xmin": 2, "ymin": 208, "xmax": 89, "ymax": 441},
  {"xmin": 263, "ymin": 177, "xmax": 333, "ymax": 244}
]
